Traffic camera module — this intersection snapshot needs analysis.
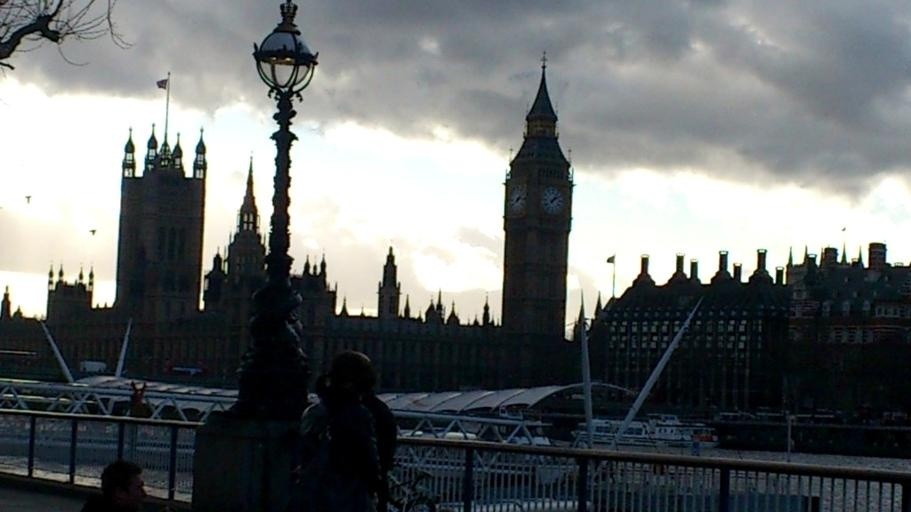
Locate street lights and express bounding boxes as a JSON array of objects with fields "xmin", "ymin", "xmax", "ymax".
[{"xmin": 238, "ymin": 2, "xmax": 323, "ymax": 503}]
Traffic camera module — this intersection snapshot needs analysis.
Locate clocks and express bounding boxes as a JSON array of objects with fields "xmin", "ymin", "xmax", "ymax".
[
  {"xmin": 507, "ymin": 186, "xmax": 528, "ymax": 213},
  {"xmin": 542, "ymin": 186, "xmax": 565, "ymax": 216}
]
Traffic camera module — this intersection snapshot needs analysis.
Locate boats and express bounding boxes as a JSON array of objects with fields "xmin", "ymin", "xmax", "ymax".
[{"xmin": 572, "ymin": 415, "xmax": 724, "ymax": 448}]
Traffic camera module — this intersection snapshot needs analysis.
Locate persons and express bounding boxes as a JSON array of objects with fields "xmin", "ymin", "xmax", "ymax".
[
  {"xmin": 690, "ymin": 430, "xmax": 700, "ymax": 456},
  {"xmin": 288, "ymin": 350, "xmax": 399, "ymax": 511},
  {"xmin": 79, "ymin": 460, "xmax": 148, "ymax": 511}
]
